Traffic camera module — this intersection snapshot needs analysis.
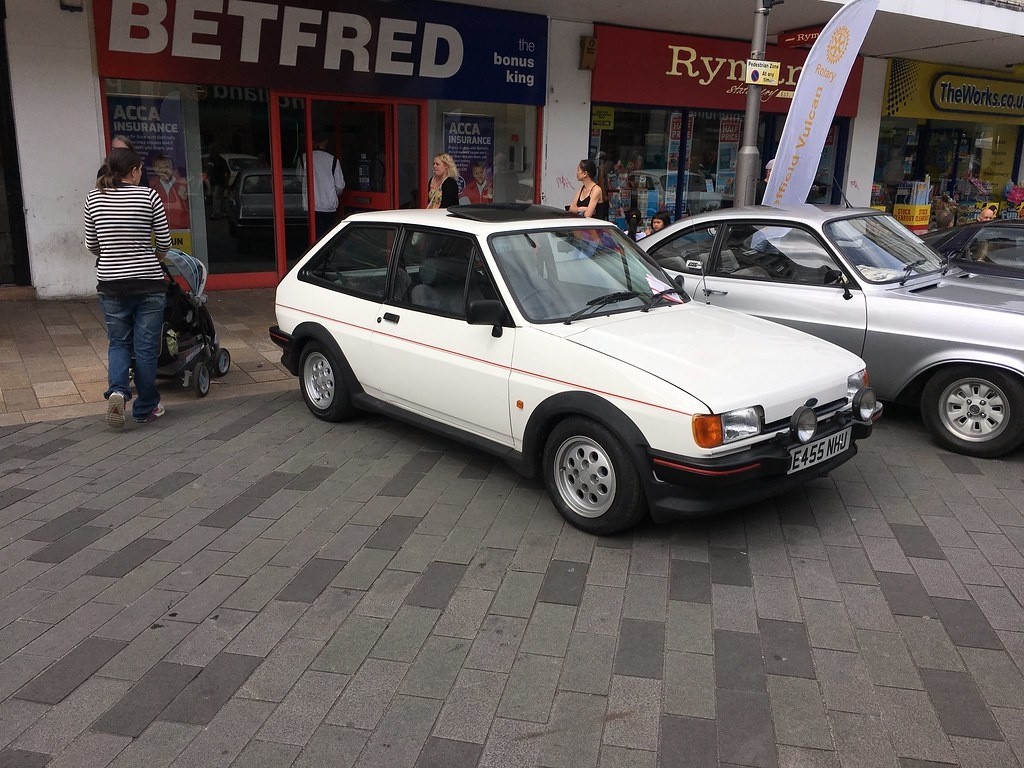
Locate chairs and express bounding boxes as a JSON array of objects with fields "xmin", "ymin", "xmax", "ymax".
[
  {"xmin": 700, "ymin": 249, "xmax": 772, "ymax": 280},
  {"xmin": 411, "ymin": 256, "xmax": 488, "ymax": 317}
]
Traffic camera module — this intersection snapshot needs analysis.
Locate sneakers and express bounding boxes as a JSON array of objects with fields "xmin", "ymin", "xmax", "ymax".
[{"xmin": 107, "ymin": 392, "xmax": 164, "ymax": 429}]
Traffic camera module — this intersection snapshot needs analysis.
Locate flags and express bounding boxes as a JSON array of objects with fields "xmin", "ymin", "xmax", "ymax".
[{"xmin": 761, "ymin": 0, "xmax": 882, "ymax": 204}]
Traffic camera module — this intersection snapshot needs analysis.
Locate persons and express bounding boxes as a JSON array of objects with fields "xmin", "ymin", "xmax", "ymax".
[
  {"xmin": 935, "ymin": 209, "xmax": 955, "ymax": 229},
  {"xmin": 755, "ymin": 159, "xmax": 776, "ymax": 205},
  {"xmin": 458, "ymin": 162, "xmax": 494, "ymax": 203},
  {"xmin": 206, "ymin": 144, "xmax": 231, "ymax": 221},
  {"xmin": 494, "ymin": 152, "xmax": 524, "ymax": 203},
  {"xmin": 632, "ymin": 150, "xmax": 645, "ymax": 170},
  {"xmin": 95, "ymin": 134, "xmax": 150, "ymax": 189},
  {"xmin": 569, "ymin": 160, "xmax": 608, "ymax": 222},
  {"xmin": 977, "ymin": 209, "xmax": 994, "ymax": 222},
  {"xmin": 595, "ymin": 151, "xmax": 615, "ymax": 220},
  {"xmin": 297, "ymin": 130, "xmax": 346, "ymax": 241},
  {"xmin": 623, "ymin": 208, "xmax": 646, "ymax": 241},
  {"xmin": 84, "ymin": 148, "xmax": 173, "ymax": 428},
  {"xmin": 651, "ymin": 211, "xmax": 670, "ymax": 232},
  {"xmin": 148, "ymin": 156, "xmax": 191, "ymax": 230},
  {"xmin": 428, "ymin": 153, "xmax": 461, "ymax": 209}
]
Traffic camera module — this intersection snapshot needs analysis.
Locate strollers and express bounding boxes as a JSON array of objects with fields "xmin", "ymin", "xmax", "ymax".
[{"xmin": 94, "ymin": 247, "xmax": 231, "ymax": 397}]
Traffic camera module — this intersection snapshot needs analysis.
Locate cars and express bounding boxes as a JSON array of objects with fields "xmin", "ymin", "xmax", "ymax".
[
  {"xmin": 914, "ymin": 218, "xmax": 1024, "ymax": 283},
  {"xmin": 199, "ymin": 153, "xmax": 261, "ymax": 210},
  {"xmin": 227, "ymin": 167, "xmax": 318, "ymax": 247},
  {"xmin": 612, "ymin": 169, "xmax": 734, "ymax": 220},
  {"xmin": 620, "ymin": 207, "xmax": 1024, "ymax": 459},
  {"xmin": 269, "ymin": 203, "xmax": 884, "ymax": 536}
]
